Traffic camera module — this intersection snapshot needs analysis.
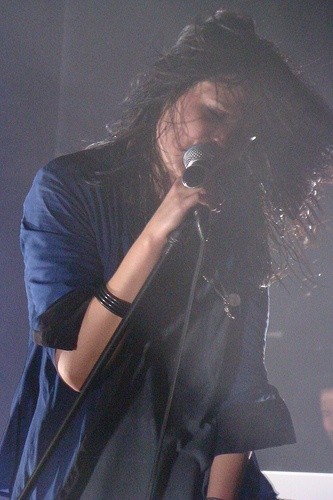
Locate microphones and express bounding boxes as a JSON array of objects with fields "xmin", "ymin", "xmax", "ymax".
[{"xmin": 183, "ymin": 143, "xmax": 215, "ymax": 239}]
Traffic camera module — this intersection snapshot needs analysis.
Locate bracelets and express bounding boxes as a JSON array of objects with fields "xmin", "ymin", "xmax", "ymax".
[
  {"xmin": 94, "ymin": 285, "xmax": 132, "ymax": 318},
  {"xmin": 206, "ymin": 497, "xmax": 222, "ymax": 500}
]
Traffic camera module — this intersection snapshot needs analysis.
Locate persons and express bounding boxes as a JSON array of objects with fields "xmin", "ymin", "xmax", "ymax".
[
  {"xmin": 319, "ymin": 389, "xmax": 333, "ymax": 435},
  {"xmin": 0, "ymin": 10, "xmax": 333, "ymax": 500}
]
